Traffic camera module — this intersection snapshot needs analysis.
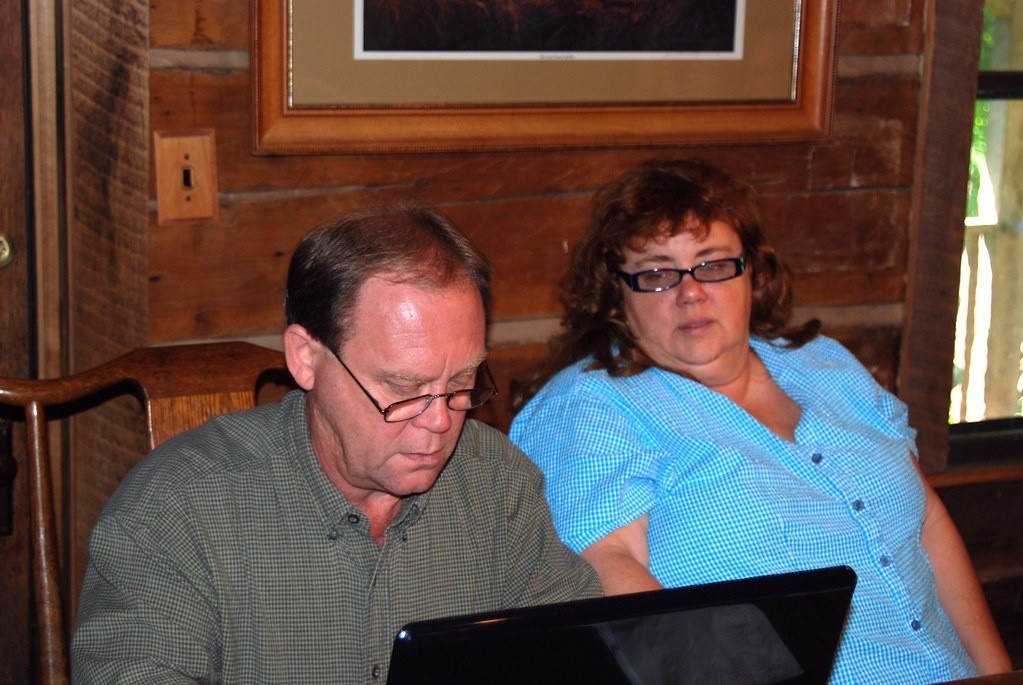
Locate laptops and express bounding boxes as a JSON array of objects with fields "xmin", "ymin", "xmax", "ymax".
[{"xmin": 383, "ymin": 565, "xmax": 858, "ymax": 685}]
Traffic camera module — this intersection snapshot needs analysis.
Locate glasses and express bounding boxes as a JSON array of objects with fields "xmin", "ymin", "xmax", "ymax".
[
  {"xmin": 611, "ymin": 250, "xmax": 747, "ymax": 292},
  {"xmin": 312, "ymin": 336, "xmax": 498, "ymax": 423}
]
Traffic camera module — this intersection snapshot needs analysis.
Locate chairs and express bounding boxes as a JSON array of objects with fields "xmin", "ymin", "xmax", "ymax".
[{"xmin": 0, "ymin": 341, "xmax": 301, "ymax": 685}]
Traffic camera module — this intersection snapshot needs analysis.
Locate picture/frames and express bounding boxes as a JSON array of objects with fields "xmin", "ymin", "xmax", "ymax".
[{"xmin": 252, "ymin": 0, "xmax": 837, "ymax": 155}]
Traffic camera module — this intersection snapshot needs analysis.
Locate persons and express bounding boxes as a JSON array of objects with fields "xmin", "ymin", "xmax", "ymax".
[
  {"xmin": 507, "ymin": 157, "xmax": 1012, "ymax": 685},
  {"xmin": 72, "ymin": 203, "xmax": 602, "ymax": 684}
]
